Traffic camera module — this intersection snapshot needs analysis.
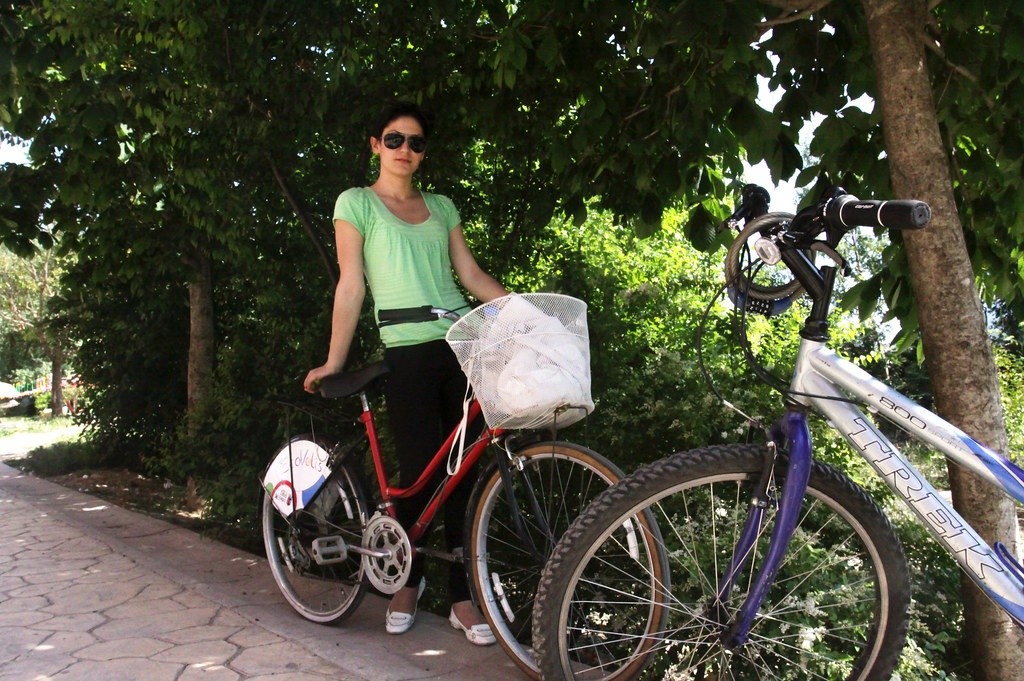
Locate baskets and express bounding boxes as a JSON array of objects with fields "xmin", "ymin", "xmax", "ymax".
[{"xmin": 445, "ymin": 292, "xmax": 595, "ymax": 430}]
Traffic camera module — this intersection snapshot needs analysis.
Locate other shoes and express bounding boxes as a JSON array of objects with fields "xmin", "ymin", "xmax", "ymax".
[
  {"xmin": 385, "ymin": 575, "xmax": 426, "ymax": 634},
  {"xmin": 449, "ymin": 604, "xmax": 496, "ymax": 644}
]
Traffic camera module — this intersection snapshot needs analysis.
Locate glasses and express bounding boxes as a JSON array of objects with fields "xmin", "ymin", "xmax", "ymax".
[{"xmin": 378, "ymin": 130, "xmax": 427, "ymax": 153}]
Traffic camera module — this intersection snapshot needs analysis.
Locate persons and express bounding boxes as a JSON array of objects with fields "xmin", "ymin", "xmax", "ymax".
[{"xmin": 303, "ymin": 100, "xmax": 510, "ymax": 646}]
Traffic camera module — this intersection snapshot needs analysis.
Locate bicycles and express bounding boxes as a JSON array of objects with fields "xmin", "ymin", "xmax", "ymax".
[
  {"xmin": 531, "ymin": 179, "xmax": 1024, "ymax": 681},
  {"xmin": 257, "ymin": 289, "xmax": 672, "ymax": 681}
]
